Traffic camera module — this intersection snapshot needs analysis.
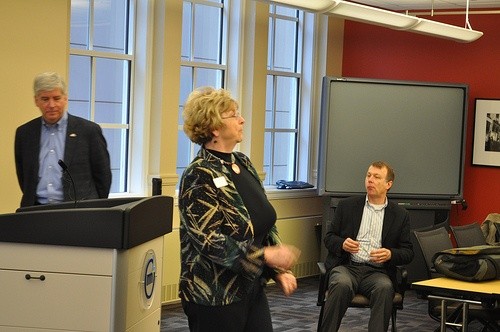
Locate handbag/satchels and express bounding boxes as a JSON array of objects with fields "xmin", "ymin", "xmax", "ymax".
[{"xmin": 434, "ymin": 244, "xmax": 500, "ymax": 282}]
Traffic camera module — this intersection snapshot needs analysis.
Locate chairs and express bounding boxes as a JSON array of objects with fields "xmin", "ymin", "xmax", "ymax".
[
  {"xmin": 486, "ymin": 221, "xmax": 500, "ymax": 243},
  {"xmin": 450, "ymin": 221, "xmax": 489, "ymax": 249},
  {"xmin": 316, "ymin": 220, "xmax": 404, "ymax": 332},
  {"xmin": 411, "ymin": 226, "xmax": 500, "ymax": 332}
]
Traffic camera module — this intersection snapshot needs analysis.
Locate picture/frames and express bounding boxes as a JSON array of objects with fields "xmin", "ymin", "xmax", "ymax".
[{"xmin": 471, "ymin": 98, "xmax": 500, "ymax": 168}]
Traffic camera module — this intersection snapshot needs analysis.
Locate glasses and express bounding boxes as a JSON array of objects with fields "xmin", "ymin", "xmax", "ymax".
[{"xmin": 222, "ymin": 112, "xmax": 241, "ymax": 119}]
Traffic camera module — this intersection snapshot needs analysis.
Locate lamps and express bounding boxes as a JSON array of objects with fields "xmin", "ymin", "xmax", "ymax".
[{"xmin": 269, "ymin": 0, "xmax": 483, "ymax": 44}]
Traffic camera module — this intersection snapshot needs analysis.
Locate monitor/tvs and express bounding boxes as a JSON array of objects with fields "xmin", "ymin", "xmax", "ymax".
[{"xmin": 317, "ymin": 76, "xmax": 469, "ymax": 203}]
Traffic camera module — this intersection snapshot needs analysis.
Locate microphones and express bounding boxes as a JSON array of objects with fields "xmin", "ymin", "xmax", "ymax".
[{"xmin": 57, "ymin": 159, "xmax": 77, "ymax": 210}]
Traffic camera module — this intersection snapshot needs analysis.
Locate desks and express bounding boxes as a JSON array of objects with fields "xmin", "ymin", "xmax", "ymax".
[{"xmin": 411, "ymin": 275, "xmax": 500, "ymax": 332}]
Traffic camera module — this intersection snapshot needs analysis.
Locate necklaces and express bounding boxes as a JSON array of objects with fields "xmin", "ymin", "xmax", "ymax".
[{"xmin": 220, "ymin": 154, "xmax": 241, "ymax": 174}]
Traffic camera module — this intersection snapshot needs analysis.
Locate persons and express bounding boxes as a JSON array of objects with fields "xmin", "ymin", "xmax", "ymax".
[
  {"xmin": 14, "ymin": 72, "xmax": 112, "ymax": 208},
  {"xmin": 177, "ymin": 87, "xmax": 302, "ymax": 332},
  {"xmin": 321, "ymin": 161, "xmax": 415, "ymax": 332}
]
